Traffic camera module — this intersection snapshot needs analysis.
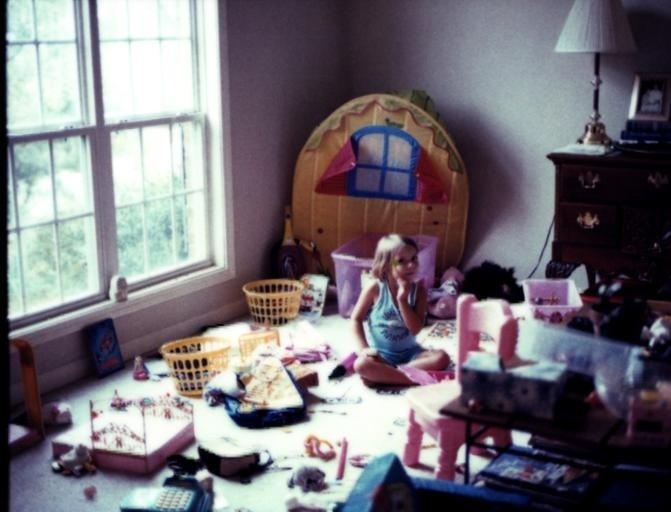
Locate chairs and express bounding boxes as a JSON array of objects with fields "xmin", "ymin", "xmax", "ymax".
[
  {"xmin": 403, "ymin": 294, "xmax": 519, "ymax": 480},
  {"xmin": 341, "ymin": 454, "xmax": 528, "ymax": 511}
]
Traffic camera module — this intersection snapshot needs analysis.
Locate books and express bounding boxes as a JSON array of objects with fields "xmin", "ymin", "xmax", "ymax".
[
  {"xmin": 83, "ymin": 317, "xmax": 126, "ymax": 380},
  {"xmin": 302, "ymin": 274, "xmax": 331, "ymax": 327},
  {"xmin": 478, "ymin": 442, "xmax": 603, "ymax": 509}
]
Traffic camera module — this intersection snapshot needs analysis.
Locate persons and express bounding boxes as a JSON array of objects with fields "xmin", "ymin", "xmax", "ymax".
[{"xmin": 350, "ymin": 234, "xmax": 451, "ymax": 387}]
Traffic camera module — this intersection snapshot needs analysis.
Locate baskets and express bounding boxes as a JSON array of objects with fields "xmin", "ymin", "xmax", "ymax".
[
  {"xmin": 241, "ymin": 278, "xmax": 305, "ymax": 327},
  {"xmin": 158, "ymin": 336, "xmax": 232, "ymax": 396},
  {"xmin": 238, "ymin": 329, "xmax": 280, "ymax": 364}
]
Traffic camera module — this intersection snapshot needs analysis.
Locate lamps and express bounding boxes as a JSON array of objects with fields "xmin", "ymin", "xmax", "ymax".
[{"xmin": 554, "ymin": 1, "xmax": 638, "ymax": 145}]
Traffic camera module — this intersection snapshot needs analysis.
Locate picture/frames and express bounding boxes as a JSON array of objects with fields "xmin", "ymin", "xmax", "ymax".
[{"xmin": 626, "ymin": 71, "xmax": 671, "ymax": 122}]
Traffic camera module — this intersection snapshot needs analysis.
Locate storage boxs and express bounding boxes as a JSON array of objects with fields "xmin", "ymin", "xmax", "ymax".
[
  {"xmin": 331, "ymin": 232, "xmax": 440, "ymax": 318},
  {"xmin": 524, "ymin": 279, "xmax": 584, "ymax": 325}
]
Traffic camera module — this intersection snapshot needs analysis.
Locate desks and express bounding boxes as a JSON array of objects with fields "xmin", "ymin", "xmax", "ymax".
[{"xmin": 438, "ymin": 300, "xmax": 671, "ymax": 484}]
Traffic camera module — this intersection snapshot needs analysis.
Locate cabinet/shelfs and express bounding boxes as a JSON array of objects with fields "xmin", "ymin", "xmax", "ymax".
[{"xmin": 547, "ymin": 146, "xmax": 670, "ymax": 300}]
